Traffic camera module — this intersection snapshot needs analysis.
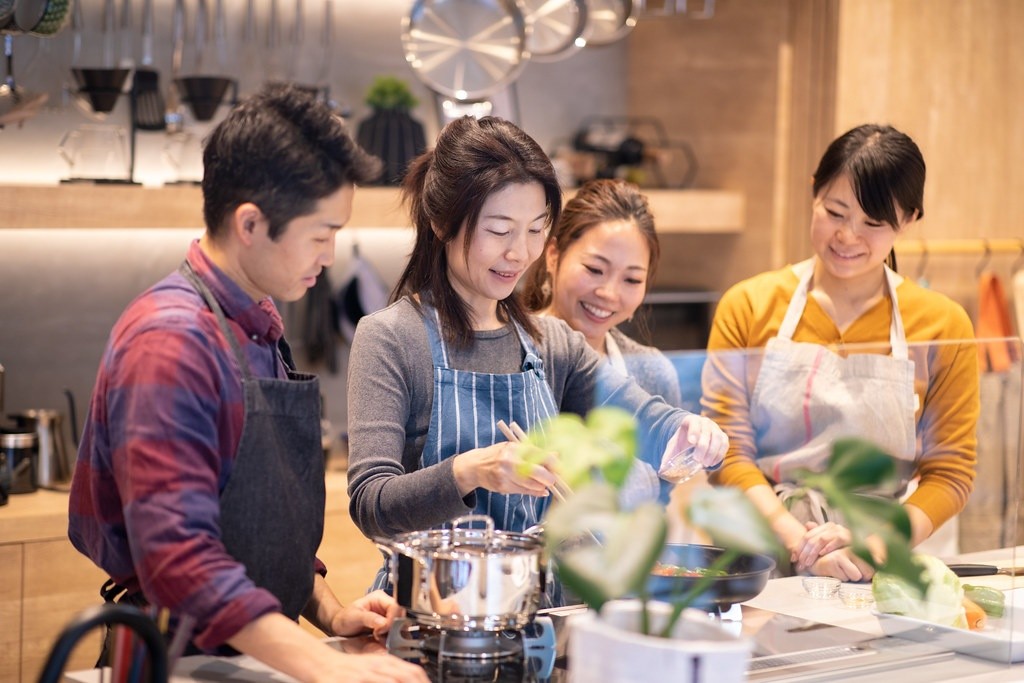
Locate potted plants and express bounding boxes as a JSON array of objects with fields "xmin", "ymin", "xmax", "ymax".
[
  {"xmin": 356, "ymin": 78, "xmax": 430, "ymax": 187},
  {"xmin": 514, "ymin": 406, "xmax": 921, "ymax": 683}
]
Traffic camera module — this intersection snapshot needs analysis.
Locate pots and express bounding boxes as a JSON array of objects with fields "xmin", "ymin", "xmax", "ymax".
[
  {"xmin": 373, "ymin": 515, "xmax": 544, "ymax": 631},
  {"xmin": 400, "ymin": 1, "xmax": 644, "ymax": 105},
  {"xmin": 626, "ymin": 541, "xmax": 777, "ymax": 607}
]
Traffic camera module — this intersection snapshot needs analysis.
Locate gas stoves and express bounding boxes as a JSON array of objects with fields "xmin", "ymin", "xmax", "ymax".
[{"xmin": 323, "ymin": 604, "xmax": 955, "ymax": 682}]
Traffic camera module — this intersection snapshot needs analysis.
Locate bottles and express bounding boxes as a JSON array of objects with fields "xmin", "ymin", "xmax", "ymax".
[{"xmin": 1, "ymin": 432, "xmax": 37, "ymax": 495}]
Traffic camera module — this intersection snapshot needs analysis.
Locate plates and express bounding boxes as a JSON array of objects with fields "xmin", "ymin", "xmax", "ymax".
[{"xmin": 873, "ymin": 609, "xmax": 1024, "ymax": 663}]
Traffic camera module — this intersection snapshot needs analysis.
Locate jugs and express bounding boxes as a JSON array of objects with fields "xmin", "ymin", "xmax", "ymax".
[{"xmin": 6, "ymin": 399, "xmax": 70, "ymax": 487}]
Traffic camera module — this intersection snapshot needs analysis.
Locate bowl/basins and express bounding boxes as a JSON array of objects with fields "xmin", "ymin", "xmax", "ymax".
[
  {"xmin": 657, "ymin": 445, "xmax": 703, "ymax": 484},
  {"xmin": 837, "ymin": 587, "xmax": 874, "ymax": 611},
  {"xmin": 801, "ymin": 576, "xmax": 840, "ymax": 598}
]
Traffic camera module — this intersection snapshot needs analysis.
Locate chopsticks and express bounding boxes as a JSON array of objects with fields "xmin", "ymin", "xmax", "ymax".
[{"xmin": 496, "ymin": 419, "xmax": 600, "ymax": 548}]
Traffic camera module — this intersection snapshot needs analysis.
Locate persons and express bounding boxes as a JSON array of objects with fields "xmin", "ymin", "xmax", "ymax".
[
  {"xmin": 701, "ymin": 124, "xmax": 982, "ymax": 583},
  {"xmin": 347, "ymin": 116, "xmax": 730, "ymax": 606},
  {"xmin": 68, "ymin": 89, "xmax": 433, "ymax": 683},
  {"xmin": 522, "ymin": 179, "xmax": 681, "ymax": 408}
]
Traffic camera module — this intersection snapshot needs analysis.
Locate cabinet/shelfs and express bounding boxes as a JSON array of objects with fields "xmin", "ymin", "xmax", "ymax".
[
  {"xmin": 0, "ymin": 516, "xmax": 383, "ymax": 683},
  {"xmin": 628, "ymin": 1, "xmax": 1024, "ymax": 292}
]
